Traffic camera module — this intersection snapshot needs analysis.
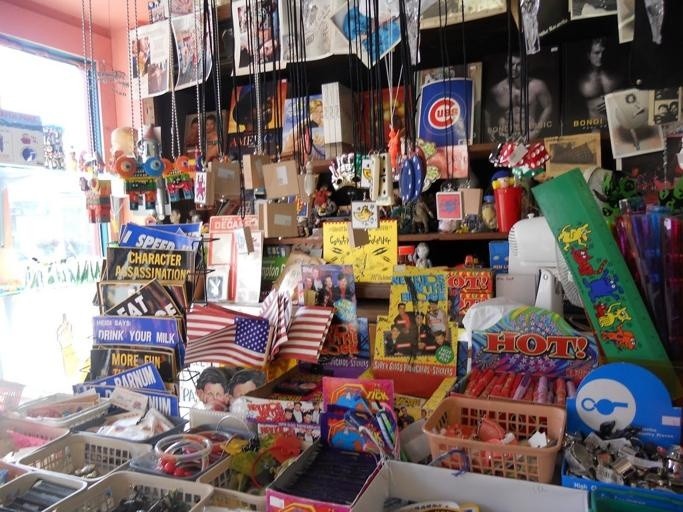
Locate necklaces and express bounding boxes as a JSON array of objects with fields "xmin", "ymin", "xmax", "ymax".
[{"xmin": 76, "ymin": 0, "xmax": 553, "ymax": 221}]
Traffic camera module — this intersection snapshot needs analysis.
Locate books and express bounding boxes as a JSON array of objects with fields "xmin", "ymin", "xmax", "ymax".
[{"xmin": 67, "ymin": 214, "xmax": 494, "ymax": 460}]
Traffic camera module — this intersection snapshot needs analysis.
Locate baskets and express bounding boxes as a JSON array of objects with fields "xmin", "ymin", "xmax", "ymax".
[
  {"xmin": 0, "ymin": 392, "xmax": 311, "ymax": 512},
  {"xmin": 420, "ymin": 396, "xmax": 566, "ymax": 484}
]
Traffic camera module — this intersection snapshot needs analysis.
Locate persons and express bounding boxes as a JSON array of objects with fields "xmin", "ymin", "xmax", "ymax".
[
  {"xmin": 654, "ymin": 104, "xmax": 673, "ymax": 124},
  {"xmin": 624, "ymin": 94, "xmax": 645, "ymax": 153},
  {"xmin": 573, "ymin": 37, "xmax": 622, "ymax": 134},
  {"xmin": 667, "ymin": 102, "xmax": 678, "ymax": 120}
]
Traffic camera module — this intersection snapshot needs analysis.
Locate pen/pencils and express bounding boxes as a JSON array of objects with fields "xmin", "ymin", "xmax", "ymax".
[{"xmin": 374, "ymin": 399, "xmax": 410, "ymax": 463}]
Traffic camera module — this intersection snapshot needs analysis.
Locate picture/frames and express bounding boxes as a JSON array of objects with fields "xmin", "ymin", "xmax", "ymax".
[{"xmin": 435, "ymin": 191, "xmax": 463, "ymax": 221}]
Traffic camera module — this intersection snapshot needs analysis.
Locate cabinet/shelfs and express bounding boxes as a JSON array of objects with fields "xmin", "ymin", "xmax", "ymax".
[{"xmin": 260, "ymin": 137, "xmax": 551, "ymax": 246}]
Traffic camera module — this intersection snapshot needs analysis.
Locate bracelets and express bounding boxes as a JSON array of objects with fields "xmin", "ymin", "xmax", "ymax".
[{"xmin": 145, "ymin": 425, "xmax": 240, "ymax": 477}]
